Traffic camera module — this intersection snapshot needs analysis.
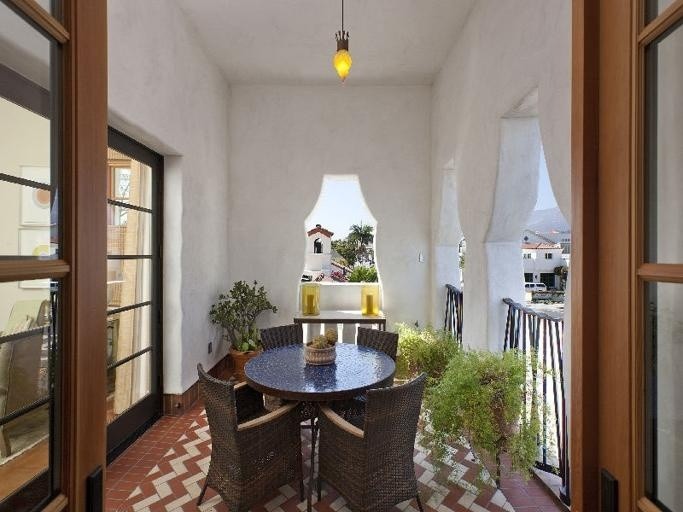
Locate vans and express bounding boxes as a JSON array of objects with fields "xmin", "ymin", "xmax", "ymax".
[{"xmin": 525, "ymin": 283, "xmax": 547, "ymax": 293}]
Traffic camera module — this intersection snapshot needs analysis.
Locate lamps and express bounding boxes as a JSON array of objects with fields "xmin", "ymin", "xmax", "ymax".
[{"xmin": 332, "ymin": 0, "xmax": 353, "ymax": 82}]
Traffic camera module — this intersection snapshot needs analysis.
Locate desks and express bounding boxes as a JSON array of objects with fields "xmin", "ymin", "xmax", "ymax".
[
  {"xmin": 293, "ymin": 310, "xmax": 386, "ymax": 343},
  {"xmin": 240, "ymin": 341, "xmax": 398, "ymax": 508}
]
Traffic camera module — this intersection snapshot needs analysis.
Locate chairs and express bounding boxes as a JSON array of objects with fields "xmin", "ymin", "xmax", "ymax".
[
  {"xmin": 195, "ymin": 361, "xmax": 305, "ymax": 508},
  {"xmin": 258, "ymin": 324, "xmax": 306, "ymax": 351},
  {"xmin": 315, "ymin": 370, "xmax": 424, "ymax": 506},
  {"xmin": 356, "ymin": 325, "xmax": 400, "ymax": 361}
]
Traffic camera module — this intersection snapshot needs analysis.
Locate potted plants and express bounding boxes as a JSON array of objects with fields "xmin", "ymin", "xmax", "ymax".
[{"xmin": 208, "ymin": 279, "xmax": 278, "ymax": 377}]
